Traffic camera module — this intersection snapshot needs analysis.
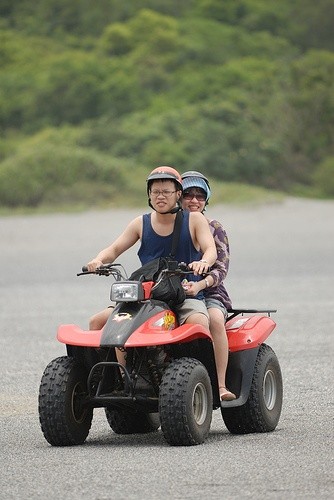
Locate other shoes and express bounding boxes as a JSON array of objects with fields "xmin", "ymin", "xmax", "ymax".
[{"xmin": 219, "ymin": 386, "xmax": 238, "ymax": 402}]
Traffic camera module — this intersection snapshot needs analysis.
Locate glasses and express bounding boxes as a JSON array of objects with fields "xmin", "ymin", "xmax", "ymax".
[
  {"xmin": 149, "ymin": 188, "xmax": 176, "ymax": 197},
  {"xmin": 182, "ymin": 192, "xmax": 207, "ymax": 201}
]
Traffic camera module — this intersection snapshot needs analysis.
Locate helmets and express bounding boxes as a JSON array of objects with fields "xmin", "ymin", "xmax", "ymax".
[
  {"xmin": 179, "ymin": 170, "xmax": 211, "ymax": 200},
  {"xmin": 145, "ymin": 166, "xmax": 184, "ymax": 192}
]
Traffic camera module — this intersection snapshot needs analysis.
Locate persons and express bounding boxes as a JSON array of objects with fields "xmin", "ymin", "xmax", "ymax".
[
  {"xmin": 115, "ymin": 170, "xmax": 237, "ymax": 400},
  {"xmin": 88, "ymin": 166, "xmax": 217, "ymax": 352}
]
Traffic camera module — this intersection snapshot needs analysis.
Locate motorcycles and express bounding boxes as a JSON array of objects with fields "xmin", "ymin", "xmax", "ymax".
[{"xmin": 38, "ymin": 262, "xmax": 283, "ymax": 447}]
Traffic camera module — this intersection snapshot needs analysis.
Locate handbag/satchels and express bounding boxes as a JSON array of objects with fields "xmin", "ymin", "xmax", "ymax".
[{"xmin": 116, "ymin": 256, "xmax": 186, "ymax": 311}]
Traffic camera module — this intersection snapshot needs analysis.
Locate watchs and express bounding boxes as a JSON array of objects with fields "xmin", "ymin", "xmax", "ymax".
[
  {"xmin": 197, "ymin": 259, "xmax": 208, "ymax": 263},
  {"xmin": 206, "ymin": 278, "xmax": 210, "ymax": 289}
]
